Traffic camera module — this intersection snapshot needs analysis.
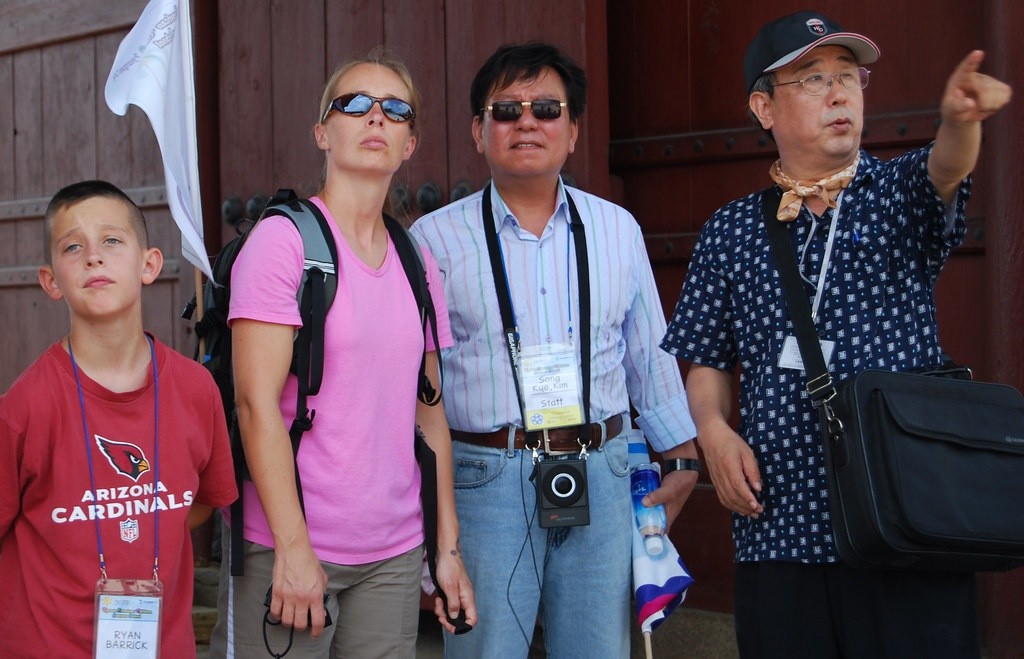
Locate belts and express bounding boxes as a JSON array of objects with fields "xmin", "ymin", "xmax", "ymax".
[{"xmin": 448, "ymin": 414, "xmax": 623, "ymax": 457}]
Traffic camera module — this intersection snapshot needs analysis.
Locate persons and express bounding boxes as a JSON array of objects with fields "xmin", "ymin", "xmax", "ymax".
[
  {"xmin": 1, "ymin": 179, "xmax": 240, "ymax": 659},
  {"xmin": 660, "ymin": 12, "xmax": 1013, "ymax": 659},
  {"xmin": 407, "ymin": 41, "xmax": 701, "ymax": 659},
  {"xmin": 210, "ymin": 48, "xmax": 480, "ymax": 659}
]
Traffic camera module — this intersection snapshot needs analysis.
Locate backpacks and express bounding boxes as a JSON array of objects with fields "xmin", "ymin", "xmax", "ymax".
[{"xmin": 181, "ymin": 189, "xmax": 444, "ymax": 481}]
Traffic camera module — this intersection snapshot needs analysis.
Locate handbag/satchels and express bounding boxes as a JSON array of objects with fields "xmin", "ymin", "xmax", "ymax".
[{"xmin": 812, "ymin": 364, "xmax": 1024, "ymax": 571}]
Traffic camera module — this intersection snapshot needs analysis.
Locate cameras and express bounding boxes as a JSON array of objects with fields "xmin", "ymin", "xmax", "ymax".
[{"xmin": 536, "ymin": 460, "xmax": 590, "ymax": 527}]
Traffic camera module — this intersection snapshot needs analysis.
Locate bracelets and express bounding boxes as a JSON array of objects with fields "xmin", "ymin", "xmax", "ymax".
[{"xmin": 660, "ymin": 457, "xmax": 700, "ymax": 472}]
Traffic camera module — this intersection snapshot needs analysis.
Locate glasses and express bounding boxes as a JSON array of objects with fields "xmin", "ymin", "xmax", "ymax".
[
  {"xmin": 321, "ymin": 93, "xmax": 416, "ymax": 130},
  {"xmin": 769, "ymin": 66, "xmax": 871, "ymax": 96},
  {"xmin": 475, "ymin": 98, "xmax": 569, "ymax": 122}
]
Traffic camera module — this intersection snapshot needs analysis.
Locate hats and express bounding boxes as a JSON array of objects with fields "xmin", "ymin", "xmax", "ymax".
[{"xmin": 743, "ymin": 11, "xmax": 882, "ymax": 94}]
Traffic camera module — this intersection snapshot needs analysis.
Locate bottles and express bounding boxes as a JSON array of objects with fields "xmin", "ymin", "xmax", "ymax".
[{"xmin": 631, "ymin": 463, "xmax": 666, "ymax": 555}]
